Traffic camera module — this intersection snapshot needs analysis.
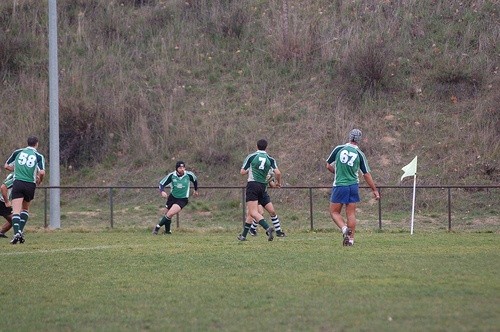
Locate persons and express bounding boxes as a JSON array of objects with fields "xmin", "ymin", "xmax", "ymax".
[
  {"xmin": 249, "ymin": 154, "xmax": 287, "ymax": 237},
  {"xmin": 4, "ymin": 135, "xmax": 46, "ymax": 245},
  {"xmin": 237, "ymin": 138, "xmax": 282, "ymax": 242},
  {"xmin": 0, "ymin": 171, "xmax": 41, "ymax": 238},
  {"xmin": 152, "ymin": 161, "xmax": 198, "ymax": 235},
  {"xmin": 325, "ymin": 129, "xmax": 381, "ymax": 247}
]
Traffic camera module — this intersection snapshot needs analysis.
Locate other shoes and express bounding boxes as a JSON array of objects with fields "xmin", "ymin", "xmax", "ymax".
[
  {"xmin": 343, "ymin": 228, "xmax": 352, "ymax": 247},
  {"xmin": 164, "ymin": 227, "xmax": 172, "ymax": 234},
  {"xmin": 276, "ymin": 232, "xmax": 285, "ymax": 238},
  {"xmin": 152, "ymin": 225, "xmax": 160, "ymax": 234},
  {"xmin": 237, "ymin": 235, "xmax": 246, "ymax": 241},
  {"xmin": 0, "ymin": 233, "xmax": 8, "ymax": 238},
  {"xmin": 9, "ymin": 233, "xmax": 21, "ymax": 245},
  {"xmin": 19, "ymin": 231, "xmax": 25, "ymax": 243},
  {"xmin": 249, "ymin": 227, "xmax": 256, "ymax": 236},
  {"xmin": 267, "ymin": 226, "xmax": 275, "ymax": 241},
  {"xmin": 349, "ymin": 238, "xmax": 354, "ymax": 246}
]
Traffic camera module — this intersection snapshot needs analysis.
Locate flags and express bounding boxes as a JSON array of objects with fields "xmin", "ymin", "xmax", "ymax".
[{"xmin": 400, "ymin": 155, "xmax": 418, "ymax": 182}]
